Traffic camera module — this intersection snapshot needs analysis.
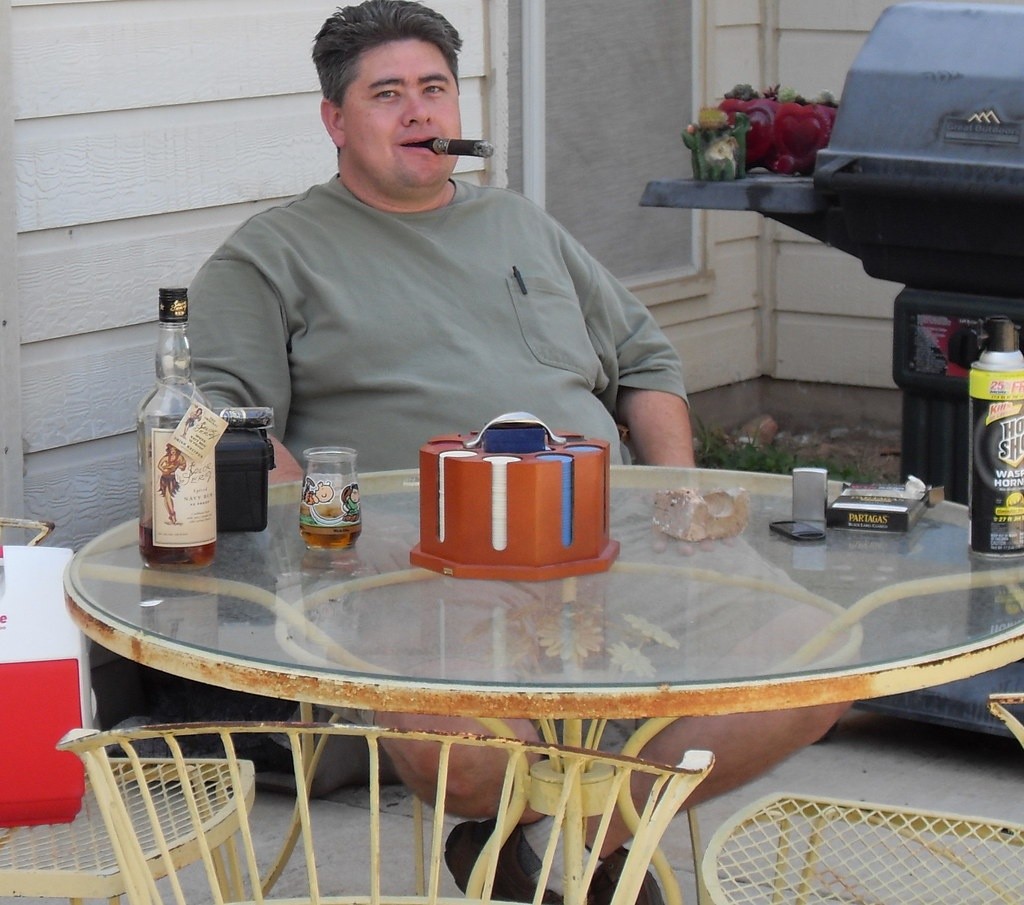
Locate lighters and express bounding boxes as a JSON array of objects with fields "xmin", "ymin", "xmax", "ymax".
[{"xmin": 791, "ymin": 468, "xmax": 828, "ymax": 521}]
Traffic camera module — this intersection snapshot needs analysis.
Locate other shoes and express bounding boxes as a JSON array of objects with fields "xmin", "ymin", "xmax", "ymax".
[
  {"xmin": 586, "ymin": 845, "xmax": 665, "ymax": 905},
  {"xmin": 443, "ymin": 818, "xmax": 564, "ymax": 905}
]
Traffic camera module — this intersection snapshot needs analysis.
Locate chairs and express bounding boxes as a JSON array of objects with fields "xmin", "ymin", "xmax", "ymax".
[
  {"xmin": 54, "ymin": 722, "xmax": 717, "ymax": 905},
  {"xmin": 0, "ymin": 756, "xmax": 258, "ymax": 905},
  {"xmin": 697, "ymin": 689, "xmax": 1024, "ymax": 905}
]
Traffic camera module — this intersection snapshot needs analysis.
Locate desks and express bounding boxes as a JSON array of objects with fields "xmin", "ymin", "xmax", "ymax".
[
  {"xmin": 637, "ymin": 179, "xmax": 1024, "ymax": 736},
  {"xmin": 61, "ymin": 465, "xmax": 1024, "ymax": 905}
]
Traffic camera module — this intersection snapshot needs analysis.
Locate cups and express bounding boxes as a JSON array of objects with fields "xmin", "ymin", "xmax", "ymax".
[{"xmin": 299, "ymin": 446, "xmax": 363, "ymax": 549}]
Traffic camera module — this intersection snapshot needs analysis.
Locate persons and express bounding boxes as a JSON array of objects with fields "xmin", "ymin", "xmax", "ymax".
[{"xmin": 186, "ymin": 0, "xmax": 855, "ymax": 905}]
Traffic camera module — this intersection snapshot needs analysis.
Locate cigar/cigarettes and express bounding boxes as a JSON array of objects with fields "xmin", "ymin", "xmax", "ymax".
[{"xmin": 421, "ymin": 138, "xmax": 494, "ymax": 158}]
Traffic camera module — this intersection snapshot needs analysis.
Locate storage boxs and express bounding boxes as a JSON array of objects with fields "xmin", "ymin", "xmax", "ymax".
[
  {"xmin": 0, "ymin": 545, "xmax": 100, "ymax": 828},
  {"xmin": 828, "ymin": 482, "xmax": 945, "ymax": 532},
  {"xmin": 214, "ymin": 428, "xmax": 275, "ymax": 533}
]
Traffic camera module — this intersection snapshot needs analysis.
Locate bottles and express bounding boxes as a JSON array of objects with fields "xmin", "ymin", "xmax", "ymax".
[
  {"xmin": 134, "ymin": 286, "xmax": 216, "ymax": 567},
  {"xmin": 967, "ymin": 314, "xmax": 1024, "ymax": 558}
]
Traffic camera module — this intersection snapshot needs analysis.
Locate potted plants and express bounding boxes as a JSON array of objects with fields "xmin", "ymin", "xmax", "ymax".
[
  {"xmin": 680, "ymin": 108, "xmax": 751, "ymax": 181},
  {"xmin": 718, "ymin": 82, "xmax": 781, "ymax": 171},
  {"xmin": 773, "ymin": 87, "xmax": 838, "ymax": 176}
]
